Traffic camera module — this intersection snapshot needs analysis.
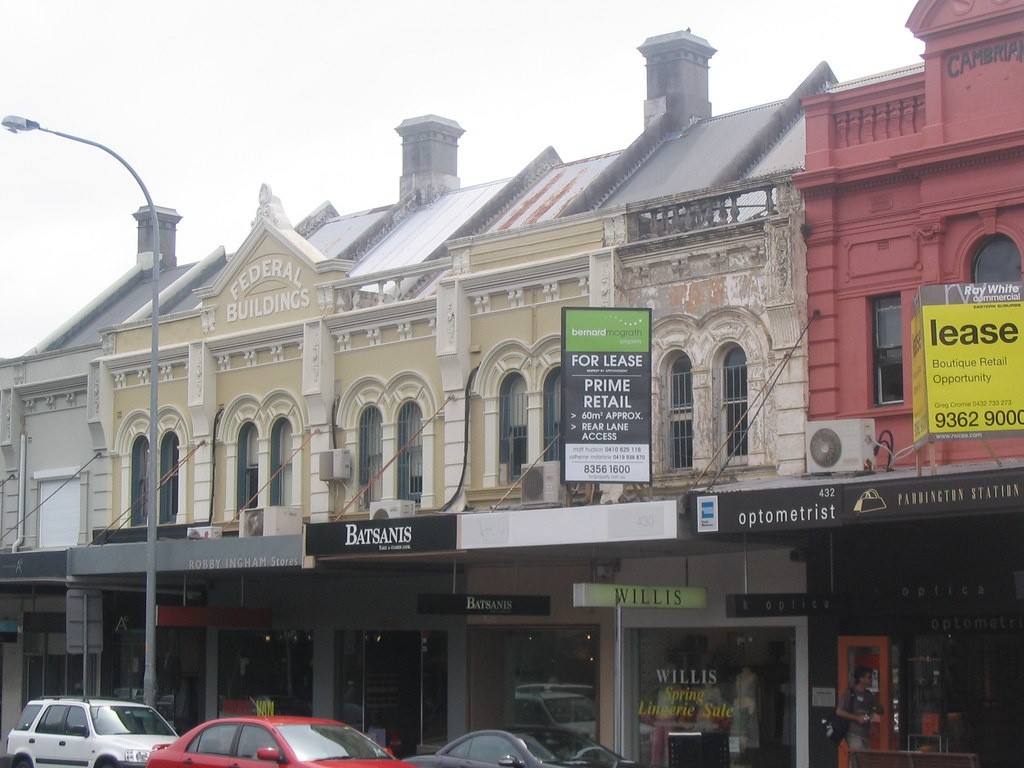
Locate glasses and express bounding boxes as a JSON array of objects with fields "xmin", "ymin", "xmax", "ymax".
[{"xmin": 862, "ymin": 674, "xmax": 872, "ymax": 679}]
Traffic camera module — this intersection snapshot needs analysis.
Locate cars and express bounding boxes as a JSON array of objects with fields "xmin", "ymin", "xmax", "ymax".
[
  {"xmin": 145, "ymin": 715, "xmax": 419, "ymax": 768},
  {"xmin": 404, "ymin": 727, "xmax": 640, "ymax": 767}
]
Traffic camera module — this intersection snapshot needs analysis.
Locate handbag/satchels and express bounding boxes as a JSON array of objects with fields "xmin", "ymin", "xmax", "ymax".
[{"xmin": 822, "ymin": 712, "xmax": 850, "ymax": 746}]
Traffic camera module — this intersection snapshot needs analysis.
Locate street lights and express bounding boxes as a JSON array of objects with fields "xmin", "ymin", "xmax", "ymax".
[{"xmin": 0, "ymin": 113, "xmax": 161, "ymax": 713}]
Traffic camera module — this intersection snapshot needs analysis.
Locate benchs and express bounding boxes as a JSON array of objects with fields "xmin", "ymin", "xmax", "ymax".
[{"xmin": 848, "ymin": 749, "xmax": 981, "ymax": 768}]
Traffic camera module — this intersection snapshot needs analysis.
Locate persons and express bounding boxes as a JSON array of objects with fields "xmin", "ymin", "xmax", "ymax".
[
  {"xmin": 640, "ymin": 661, "xmax": 765, "ymax": 768},
  {"xmin": 835, "ymin": 666, "xmax": 885, "ymax": 768}
]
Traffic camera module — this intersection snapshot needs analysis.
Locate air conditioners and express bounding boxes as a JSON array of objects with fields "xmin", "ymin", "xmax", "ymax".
[
  {"xmin": 369, "ymin": 500, "xmax": 416, "ymax": 520},
  {"xmin": 318, "ymin": 449, "xmax": 350, "ymax": 491},
  {"xmin": 804, "ymin": 420, "xmax": 876, "ymax": 477},
  {"xmin": 239, "ymin": 506, "xmax": 302, "ymax": 538},
  {"xmin": 521, "ymin": 461, "xmax": 562, "ymax": 505},
  {"xmin": 187, "ymin": 526, "xmax": 220, "ymax": 538}
]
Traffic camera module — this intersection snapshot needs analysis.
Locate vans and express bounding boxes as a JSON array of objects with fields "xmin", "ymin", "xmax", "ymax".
[{"xmin": 6, "ymin": 696, "xmax": 181, "ymax": 768}]
